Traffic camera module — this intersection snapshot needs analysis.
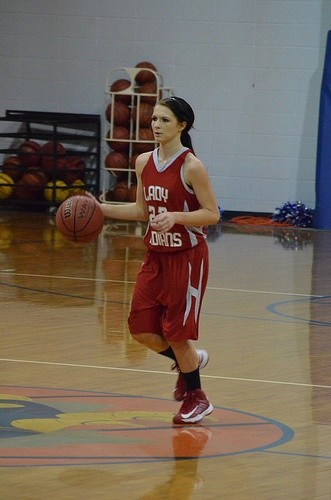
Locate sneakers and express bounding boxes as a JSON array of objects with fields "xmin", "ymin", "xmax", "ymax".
[
  {"xmin": 170, "ymin": 389, "xmax": 214, "ymax": 427},
  {"xmin": 171, "ymin": 350, "xmax": 210, "ymax": 400}
]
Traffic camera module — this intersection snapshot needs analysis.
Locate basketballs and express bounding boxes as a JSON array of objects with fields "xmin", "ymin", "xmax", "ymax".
[
  {"xmin": 0, "ymin": 142, "xmax": 90, "ymax": 201},
  {"xmin": 104, "ymin": 62, "xmax": 160, "ymax": 204},
  {"xmin": 56, "ymin": 196, "xmax": 104, "ymax": 243}
]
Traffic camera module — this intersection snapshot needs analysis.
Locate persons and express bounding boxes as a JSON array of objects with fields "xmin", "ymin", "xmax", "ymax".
[{"xmin": 86, "ymin": 96, "xmax": 220, "ymax": 426}]
